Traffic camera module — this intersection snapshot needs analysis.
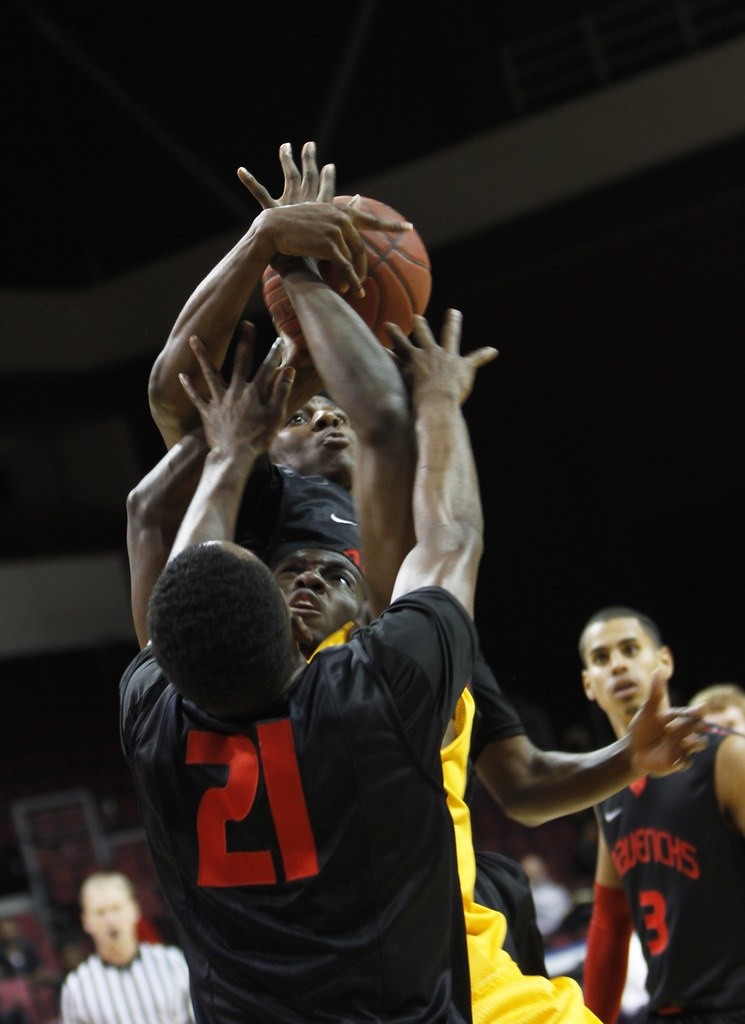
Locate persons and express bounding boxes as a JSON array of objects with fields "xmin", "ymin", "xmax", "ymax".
[{"xmin": 61, "ymin": 143, "xmax": 745, "ymax": 1024}]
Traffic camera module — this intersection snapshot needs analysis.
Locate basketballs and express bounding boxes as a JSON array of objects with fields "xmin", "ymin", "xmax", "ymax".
[{"xmin": 263, "ymin": 194, "xmax": 432, "ymax": 351}]
